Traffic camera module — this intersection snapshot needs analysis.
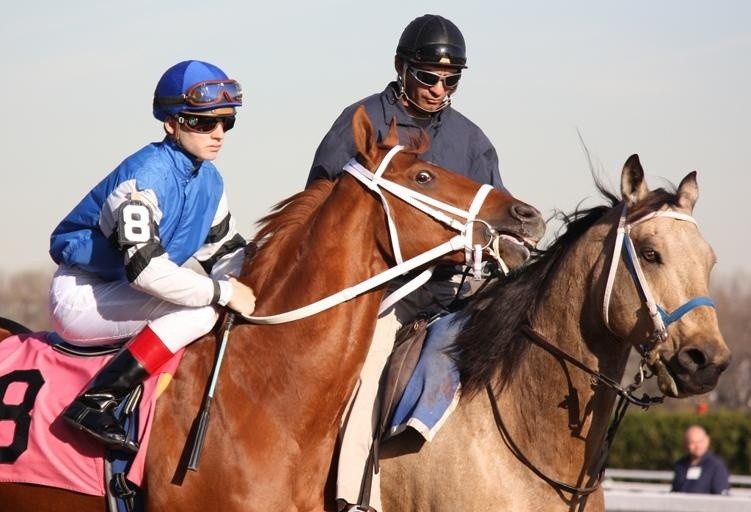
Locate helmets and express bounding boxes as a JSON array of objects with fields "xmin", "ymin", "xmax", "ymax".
[
  {"xmin": 396, "ymin": 14, "xmax": 467, "ymax": 68},
  {"xmin": 154, "ymin": 60, "xmax": 243, "ymax": 122}
]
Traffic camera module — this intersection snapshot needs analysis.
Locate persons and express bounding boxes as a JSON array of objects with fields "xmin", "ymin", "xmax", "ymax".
[
  {"xmin": 670, "ymin": 425, "xmax": 733, "ymax": 497},
  {"xmin": 305, "ymin": 13, "xmax": 514, "ymax": 511},
  {"xmin": 47, "ymin": 58, "xmax": 257, "ymax": 456}
]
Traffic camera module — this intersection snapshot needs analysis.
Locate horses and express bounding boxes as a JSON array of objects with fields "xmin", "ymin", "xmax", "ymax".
[
  {"xmin": 0, "ymin": 102, "xmax": 548, "ymax": 512},
  {"xmin": 371, "ymin": 128, "xmax": 736, "ymax": 512}
]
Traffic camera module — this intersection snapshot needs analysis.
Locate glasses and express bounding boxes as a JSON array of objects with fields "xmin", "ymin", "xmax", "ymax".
[
  {"xmin": 415, "ymin": 45, "xmax": 465, "ymax": 62},
  {"xmin": 178, "ymin": 112, "xmax": 235, "ymax": 134},
  {"xmin": 408, "ymin": 68, "xmax": 461, "ymax": 88},
  {"xmin": 187, "ymin": 79, "xmax": 243, "ymax": 106}
]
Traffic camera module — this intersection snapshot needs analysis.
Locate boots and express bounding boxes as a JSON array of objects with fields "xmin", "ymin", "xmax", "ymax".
[{"xmin": 64, "ymin": 326, "xmax": 174, "ymax": 452}]
